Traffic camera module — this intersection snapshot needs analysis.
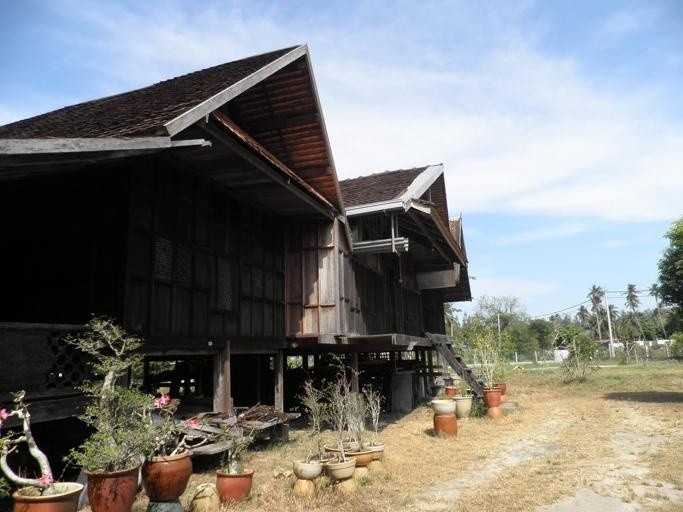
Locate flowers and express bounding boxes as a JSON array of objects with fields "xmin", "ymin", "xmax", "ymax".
[{"xmin": 0, "ymin": 388, "xmax": 63, "ymax": 496}]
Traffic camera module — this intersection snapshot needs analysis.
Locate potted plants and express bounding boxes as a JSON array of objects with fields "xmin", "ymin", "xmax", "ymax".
[
  {"xmin": 59, "ymin": 310, "xmax": 255, "ymax": 512},
  {"xmin": 292, "ymin": 351, "xmax": 384, "ymax": 480},
  {"xmin": 455, "ymin": 350, "xmax": 506, "ymax": 421}
]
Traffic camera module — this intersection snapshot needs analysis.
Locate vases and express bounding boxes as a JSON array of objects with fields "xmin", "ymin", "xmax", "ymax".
[
  {"xmin": 10, "ymin": 483, "xmax": 84, "ymax": 512},
  {"xmin": 292, "ymin": 480, "xmax": 355, "ymax": 498},
  {"xmin": 430, "ymin": 386, "xmax": 457, "ymax": 435}
]
